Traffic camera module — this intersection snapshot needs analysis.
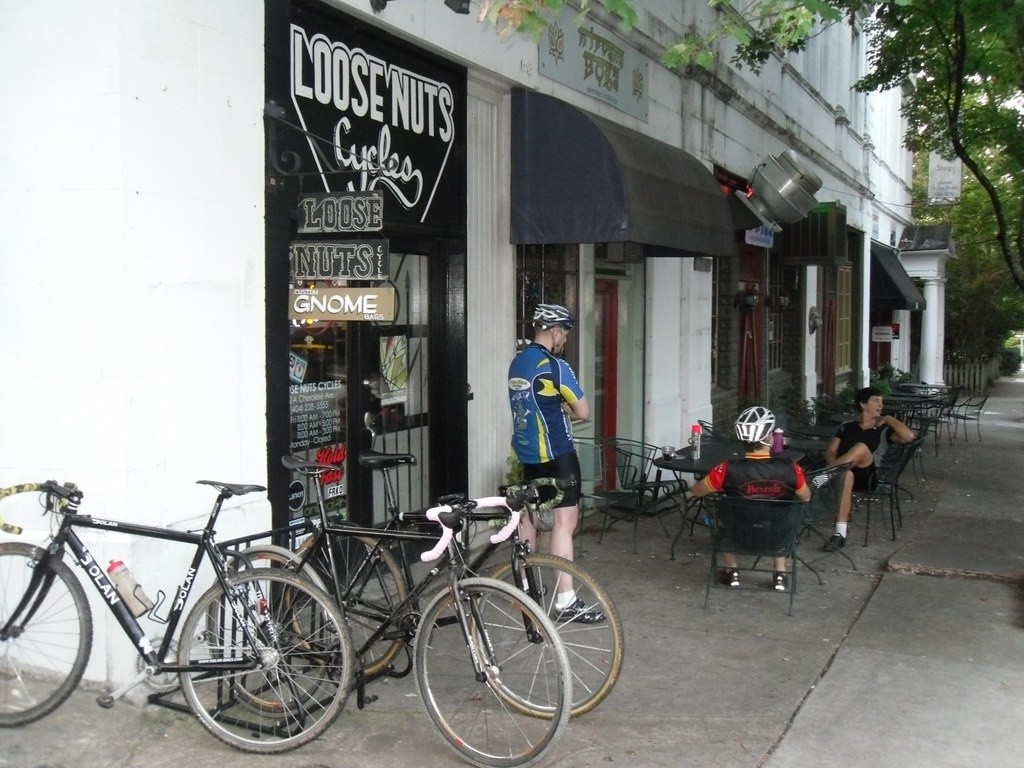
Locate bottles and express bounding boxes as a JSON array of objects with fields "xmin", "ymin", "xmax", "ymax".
[
  {"xmin": 773, "ymin": 428, "xmax": 784, "ymax": 454},
  {"xmin": 692, "ymin": 425, "xmax": 700, "ymax": 460},
  {"xmin": 106, "ymin": 559, "xmax": 151, "ymax": 617}
]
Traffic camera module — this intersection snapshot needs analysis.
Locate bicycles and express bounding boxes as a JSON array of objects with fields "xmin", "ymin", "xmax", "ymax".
[
  {"xmin": 205, "ymin": 456, "xmax": 572, "ymax": 768},
  {"xmin": 1, "ymin": 481, "xmax": 355, "ymax": 756},
  {"xmin": 282, "ymin": 454, "xmax": 626, "ymax": 720}
]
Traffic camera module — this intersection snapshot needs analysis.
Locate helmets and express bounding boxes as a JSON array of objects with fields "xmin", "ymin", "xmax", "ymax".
[
  {"xmin": 734, "ymin": 406, "xmax": 775, "ymax": 443},
  {"xmin": 532, "ymin": 303, "xmax": 575, "ymax": 330}
]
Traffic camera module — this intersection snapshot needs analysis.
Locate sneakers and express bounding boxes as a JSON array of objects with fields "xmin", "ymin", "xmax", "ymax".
[
  {"xmin": 554, "ymin": 598, "xmax": 606, "ymax": 625},
  {"xmin": 717, "ymin": 568, "xmax": 739, "ymax": 587},
  {"xmin": 773, "ymin": 573, "xmax": 788, "ymax": 592},
  {"xmin": 527, "ymin": 584, "xmax": 547, "ymax": 598}
]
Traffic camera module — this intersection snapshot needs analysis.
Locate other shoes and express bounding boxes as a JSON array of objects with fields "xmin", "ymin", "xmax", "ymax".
[
  {"xmin": 824, "ymin": 532, "xmax": 847, "ymax": 552},
  {"xmin": 813, "ymin": 471, "xmax": 829, "ymax": 489}
]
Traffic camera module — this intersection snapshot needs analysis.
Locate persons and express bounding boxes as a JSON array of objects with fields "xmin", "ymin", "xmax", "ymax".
[
  {"xmin": 509, "ymin": 303, "xmax": 607, "ymax": 622},
  {"xmin": 811, "ymin": 387, "xmax": 916, "ymax": 552},
  {"xmin": 694, "ymin": 406, "xmax": 812, "ymax": 592}
]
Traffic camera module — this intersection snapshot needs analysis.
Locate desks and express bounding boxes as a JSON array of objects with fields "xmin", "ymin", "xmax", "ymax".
[
  {"xmin": 792, "ymin": 425, "xmax": 839, "ymax": 438},
  {"xmin": 846, "ymin": 404, "xmax": 910, "ymax": 418},
  {"xmin": 791, "ymin": 437, "xmax": 852, "ymax": 527},
  {"xmin": 884, "ymin": 396, "xmax": 923, "ymax": 413},
  {"xmin": 898, "ymin": 382, "xmax": 957, "ymax": 425},
  {"xmin": 652, "ymin": 442, "xmax": 806, "ymax": 561},
  {"xmin": 830, "ymin": 412, "xmax": 857, "ymax": 423},
  {"xmin": 889, "ymin": 392, "xmax": 945, "ymax": 399}
]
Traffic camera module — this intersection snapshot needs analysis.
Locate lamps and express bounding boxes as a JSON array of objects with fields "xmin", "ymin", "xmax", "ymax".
[
  {"xmin": 766, "ymin": 287, "xmax": 789, "ymax": 313},
  {"xmin": 370, "ymin": 0, "xmax": 471, "ymax": 15},
  {"xmin": 734, "ymin": 285, "xmax": 760, "ymax": 311}
]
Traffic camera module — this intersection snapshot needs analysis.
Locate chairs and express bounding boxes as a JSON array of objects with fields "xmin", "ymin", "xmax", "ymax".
[{"xmin": 572, "ymin": 384, "xmax": 989, "ymax": 617}]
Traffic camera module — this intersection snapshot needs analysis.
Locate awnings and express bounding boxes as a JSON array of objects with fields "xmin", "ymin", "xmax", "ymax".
[
  {"xmin": 510, "ymin": 80, "xmax": 735, "ymax": 261},
  {"xmin": 870, "ymin": 242, "xmax": 926, "ymax": 313}
]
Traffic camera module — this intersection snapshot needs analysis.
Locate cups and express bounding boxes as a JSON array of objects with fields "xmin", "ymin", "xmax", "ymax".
[
  {"xmin": 783, "ymin": 437, "xmax": 792, "ymax": 451},
  {"xmin": 662, "ymin": 446, "xmax": 675, "ymax": 463}
]
